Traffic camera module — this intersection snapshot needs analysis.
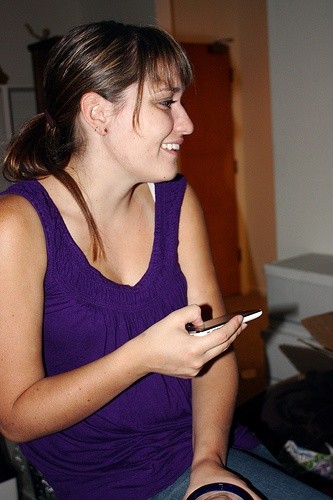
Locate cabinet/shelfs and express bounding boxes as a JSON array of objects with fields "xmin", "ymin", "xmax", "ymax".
[
  {"xmin": 263, "ymin": 253, "xmax": 333, "ymax": 386},
  {"xmin": 27, "ymin": 36, "xmax": 242, "ymax": 298}
]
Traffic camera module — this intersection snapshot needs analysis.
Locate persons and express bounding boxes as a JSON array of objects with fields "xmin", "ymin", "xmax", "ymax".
[{"xmin": 0, "ymin": 20, "xmax": 333, "ymax": 499}]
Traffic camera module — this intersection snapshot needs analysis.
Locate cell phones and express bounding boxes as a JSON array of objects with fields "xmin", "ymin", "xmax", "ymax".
[{"xmin": 185, "ymin": 309, "xmax": 263, "ymax": 336}]
